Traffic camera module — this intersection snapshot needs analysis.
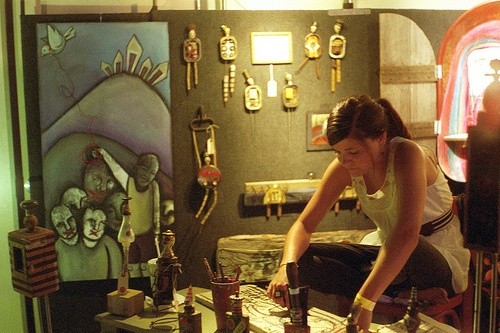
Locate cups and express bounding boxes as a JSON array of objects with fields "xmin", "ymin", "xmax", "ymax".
[{"xmin": 211, "ymin": 278, "xmax": 241, "ymax": 329}]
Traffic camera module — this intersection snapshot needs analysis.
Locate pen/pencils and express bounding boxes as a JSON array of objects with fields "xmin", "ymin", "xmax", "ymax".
[{"xmin": 202, "ymin": 258, "xmax": 240, "ymax": 283}]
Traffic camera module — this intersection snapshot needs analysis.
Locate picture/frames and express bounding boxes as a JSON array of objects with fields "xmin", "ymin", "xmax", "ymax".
[{"xmin": 250, "ymin": 31, "xmax": 294, "ymax": 65}]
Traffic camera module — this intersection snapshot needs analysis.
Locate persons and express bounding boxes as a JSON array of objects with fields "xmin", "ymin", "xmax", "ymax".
[
  {"xmin": 463, "ymin": 82, "xmax": 500, "ymax": 311},
  {"xmin": 267, "ymin": 94, "xmax": 470, "ymax": 333}
]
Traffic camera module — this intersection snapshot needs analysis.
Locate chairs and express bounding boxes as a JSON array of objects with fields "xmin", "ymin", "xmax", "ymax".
[{"xmin": 336, "ymin": 192, "xmax": 500, "ymax": 333}]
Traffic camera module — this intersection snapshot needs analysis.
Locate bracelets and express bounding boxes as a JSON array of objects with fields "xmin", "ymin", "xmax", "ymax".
[
  {"xmin": 354, "ymin": 294, "xmax": 376, "ymax": 312},
  {"xmin": 281, "ymin": 263, "xmax": 298, "ymax": 268}
]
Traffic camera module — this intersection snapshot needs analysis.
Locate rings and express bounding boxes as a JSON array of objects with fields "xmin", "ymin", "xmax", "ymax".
[{"xmin": 275, "ymin": 291, "xmax": 282, "ymax": 297}]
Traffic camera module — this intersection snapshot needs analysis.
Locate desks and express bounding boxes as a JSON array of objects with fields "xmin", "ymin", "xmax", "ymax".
[{"xmin": 94, "ymin": 286, "xmax": 256, "ymax": 333}]
